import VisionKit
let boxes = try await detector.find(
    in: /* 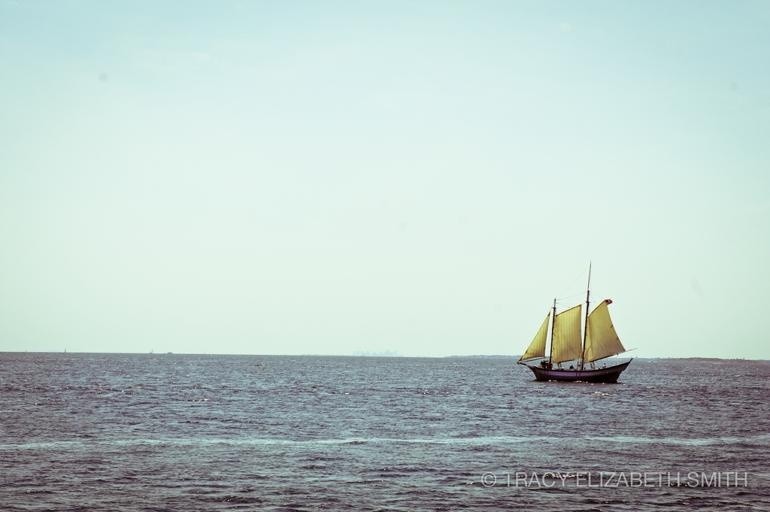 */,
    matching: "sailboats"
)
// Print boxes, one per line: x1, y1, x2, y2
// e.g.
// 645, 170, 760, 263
517, 258, 635, 383
